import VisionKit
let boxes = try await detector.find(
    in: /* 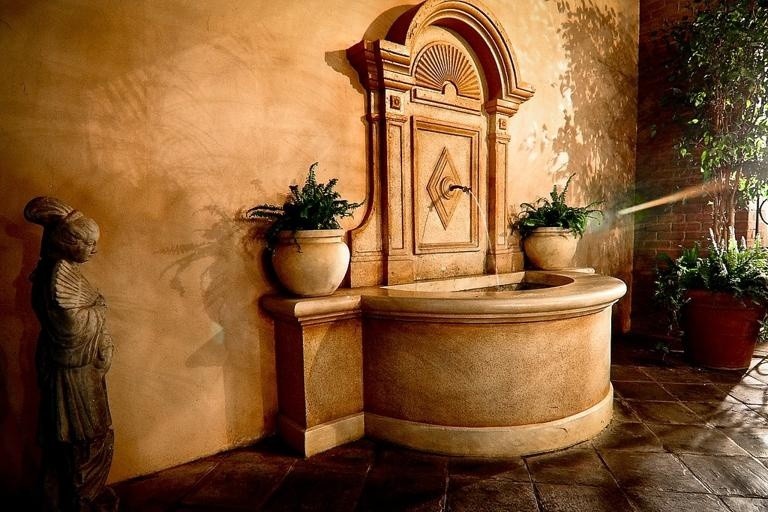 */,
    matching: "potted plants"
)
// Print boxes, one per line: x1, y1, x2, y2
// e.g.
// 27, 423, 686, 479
243, 162, 368, 298
509, 173, 608, 270
652, 232, 768, 372
